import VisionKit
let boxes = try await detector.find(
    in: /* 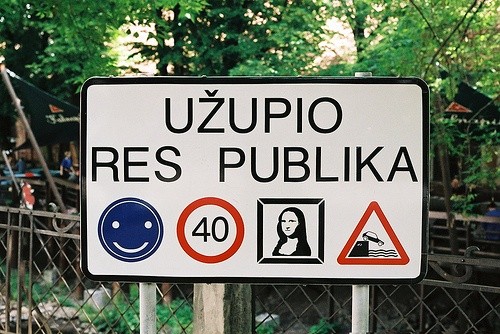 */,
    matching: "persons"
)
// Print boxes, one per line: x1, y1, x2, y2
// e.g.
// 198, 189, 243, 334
19, 179, 37, 211
58, 150, 77, 176
446, 176, 467, 227
478, 201, 500, 239
11, 156, 26, 173
